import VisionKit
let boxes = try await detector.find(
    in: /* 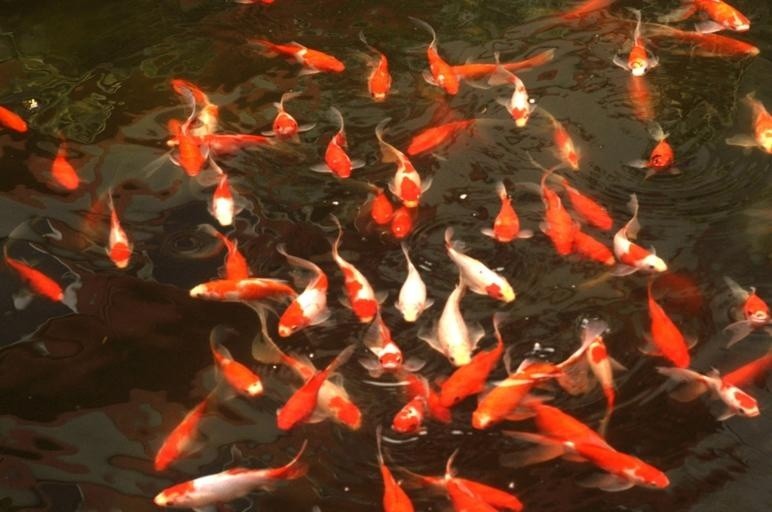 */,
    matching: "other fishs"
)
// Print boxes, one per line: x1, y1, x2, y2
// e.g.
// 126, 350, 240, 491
147, 1, 772, 512
